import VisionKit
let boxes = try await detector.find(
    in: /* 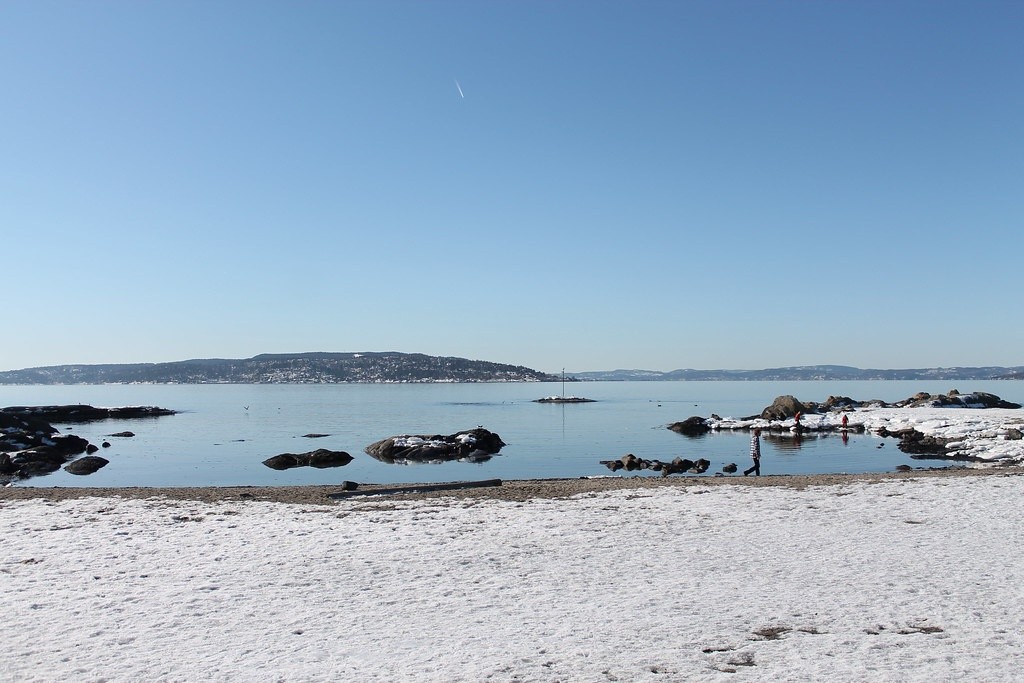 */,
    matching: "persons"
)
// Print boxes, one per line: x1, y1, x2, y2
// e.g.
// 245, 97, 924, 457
842, 414, 849, 428
795, 410, 801, 424
841, 432, 849, 446
743, 427, 762, 476
